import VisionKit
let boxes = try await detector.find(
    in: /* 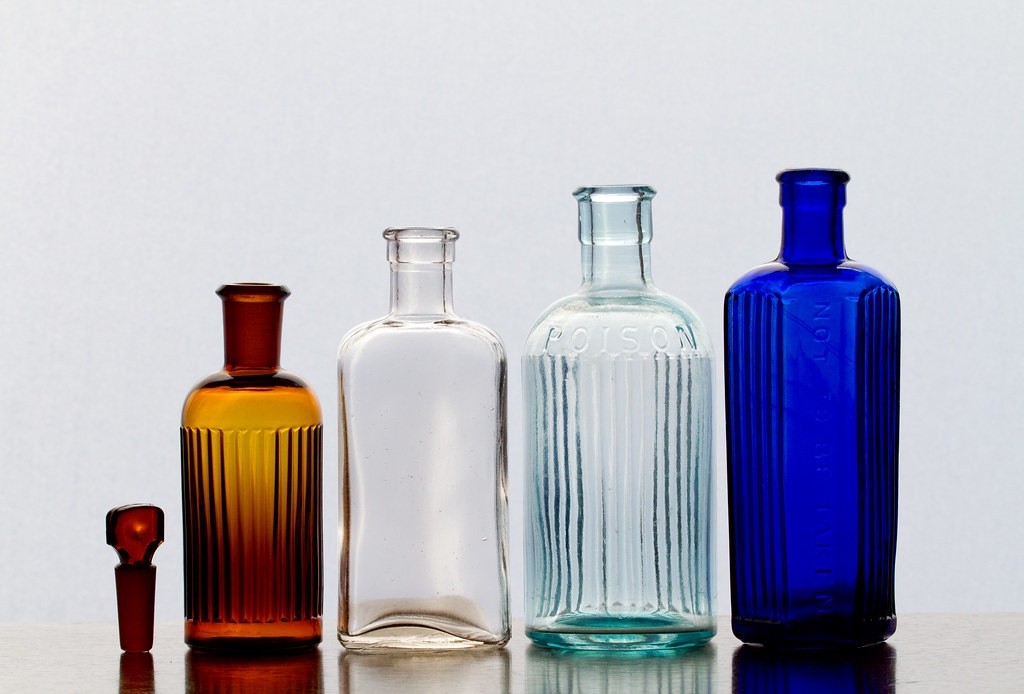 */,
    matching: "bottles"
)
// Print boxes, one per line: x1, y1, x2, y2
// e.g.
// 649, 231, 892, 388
724, 168, 902, 649
517, 184, 716, 657
178, 281, 323, 654
337, 225, 512, 660
